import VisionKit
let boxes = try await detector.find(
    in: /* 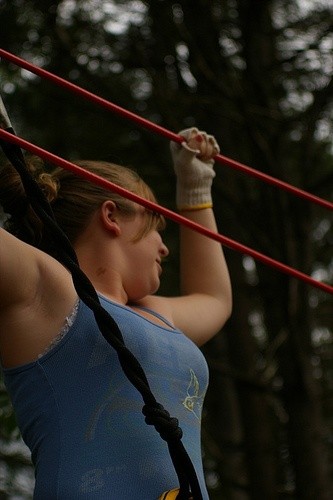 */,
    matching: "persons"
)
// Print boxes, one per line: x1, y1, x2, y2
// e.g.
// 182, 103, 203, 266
1, 128, 232, 499
